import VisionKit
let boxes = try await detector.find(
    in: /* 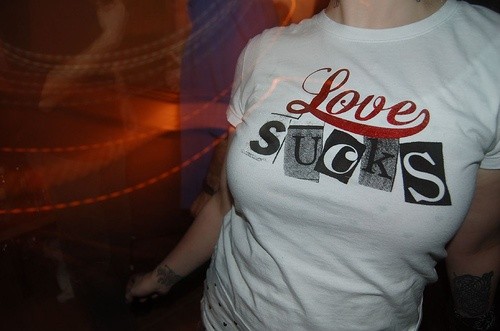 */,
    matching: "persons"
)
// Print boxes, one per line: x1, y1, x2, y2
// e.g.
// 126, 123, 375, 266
127, 0, 499, 330
